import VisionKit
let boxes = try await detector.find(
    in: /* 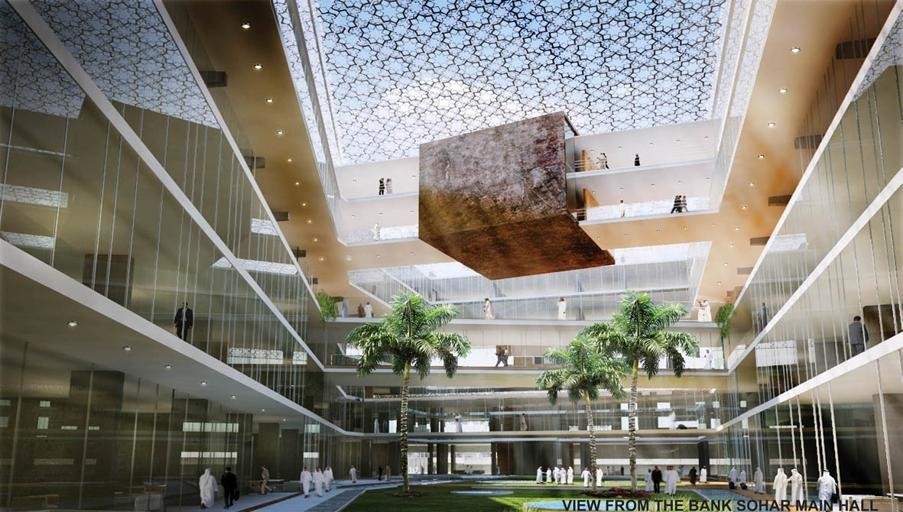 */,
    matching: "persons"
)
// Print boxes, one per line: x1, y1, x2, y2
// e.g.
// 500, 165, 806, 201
469, 465, 472, 475
502, 345, 509, 367
555, 296, 567, 320
373, 418, 380, 432
602, 152, 609, 169
376, 464, 384, 481
348, 463, 359, 483
842, 314, 868, 358
172, 300, 193, 343
675, 195, 682, 213
815, 469, 836, 511
634, 153, 640, 166
198, 467, 217, 508
322, 464, 333, 493
339, 296, 350, 318
455, 413, 464, 432
669, 195, 681, 213
751, 302, 769, 336
372, 221, 382, 240
696, 301, 704, 322
377, 177, 384, 195
617, 200, 625, 218
259, 462, 273, 495
494, 346, 507, 367
703, 349, 713, 369
299, 464, 312, 497
535, 465, 604, 488
482, 297, 494, 320
464, 464, 469, 475
596, 153, 606, 170
681, 195, 688, 213
356, 302, 367, 317
219, 465, 238, 509
728, 465, 805, 507
642, 464, 707, 496
312, 465, 326, 497
701, 299, 712, 322
519, 412, 528, 430
383, 464, 391, 481
385, 178, 392, 193
363, 301, 374, 319
